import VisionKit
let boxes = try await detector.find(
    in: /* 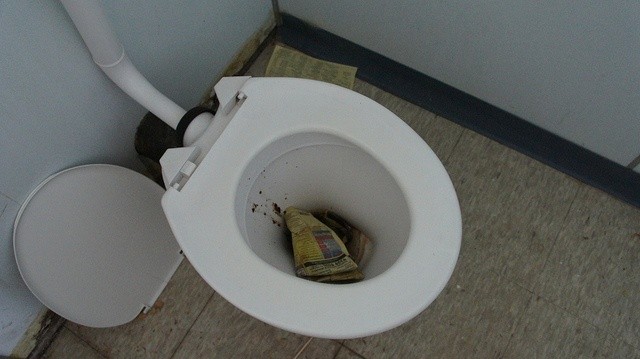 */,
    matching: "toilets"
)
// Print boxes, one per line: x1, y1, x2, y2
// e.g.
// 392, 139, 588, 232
157, 74, 464, 341
